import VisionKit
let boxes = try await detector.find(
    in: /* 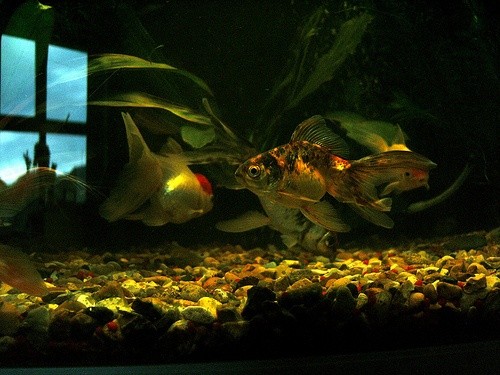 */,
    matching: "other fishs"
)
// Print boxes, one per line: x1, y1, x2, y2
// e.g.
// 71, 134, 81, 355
0, 168, 91, 218
97, 113, 216, 227
235, 116, 437, 232
0, 247, 66, 297
326, 111, 428, 196
260, 197, 340, 261
